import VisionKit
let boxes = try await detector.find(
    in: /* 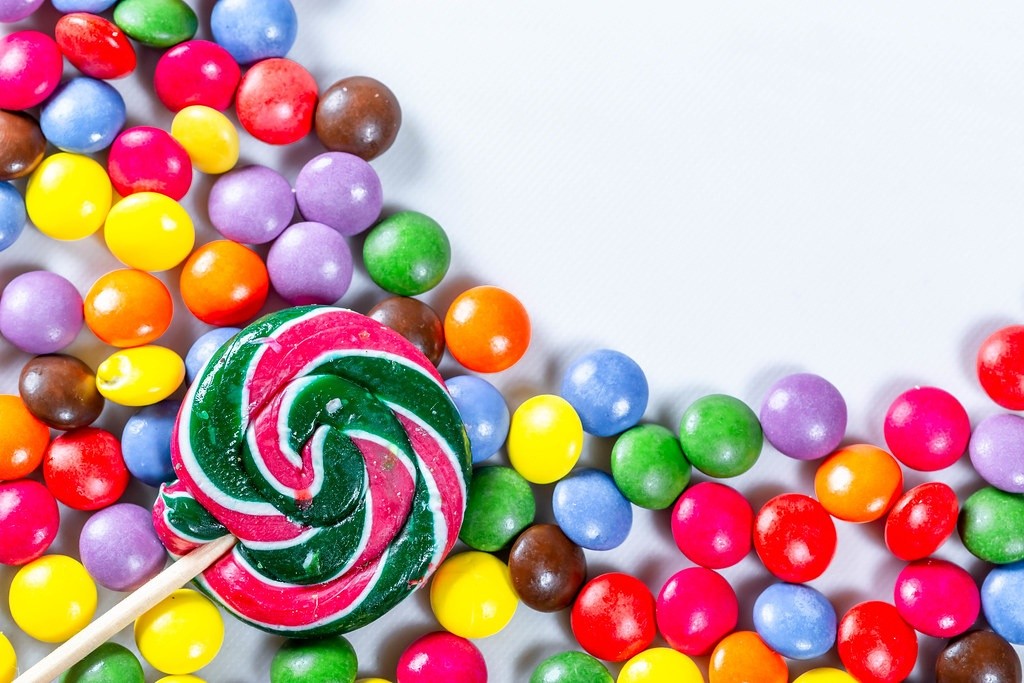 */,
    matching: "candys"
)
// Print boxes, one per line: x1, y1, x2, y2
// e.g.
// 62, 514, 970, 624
0, 0, 1024, 683
